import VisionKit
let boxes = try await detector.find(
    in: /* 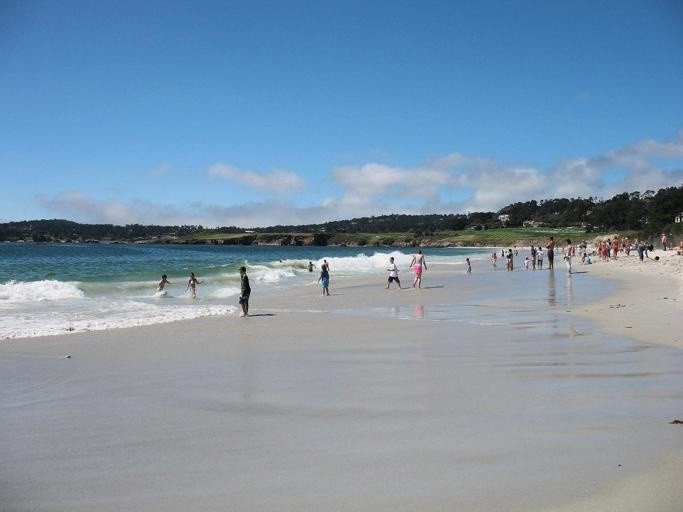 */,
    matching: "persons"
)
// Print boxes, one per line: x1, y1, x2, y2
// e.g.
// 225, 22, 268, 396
466, 258, 471, 273
158, 274, 171, 290
410, 250, 427, 289
185, 273, 203, 297
308, 259, 331, 295
386, 258, 400, 289
492, 233, 682, 274
236, 266, 251, 318
